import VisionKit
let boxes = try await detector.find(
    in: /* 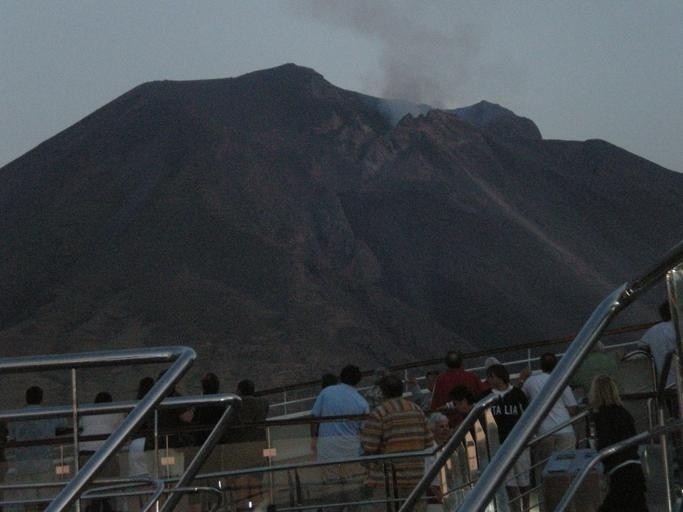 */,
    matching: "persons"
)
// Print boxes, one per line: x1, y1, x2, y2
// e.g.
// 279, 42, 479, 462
78, 371, 268, 510
636, 301, 681, 491
7, 386, 68, 511
309, 349, 648, 511
